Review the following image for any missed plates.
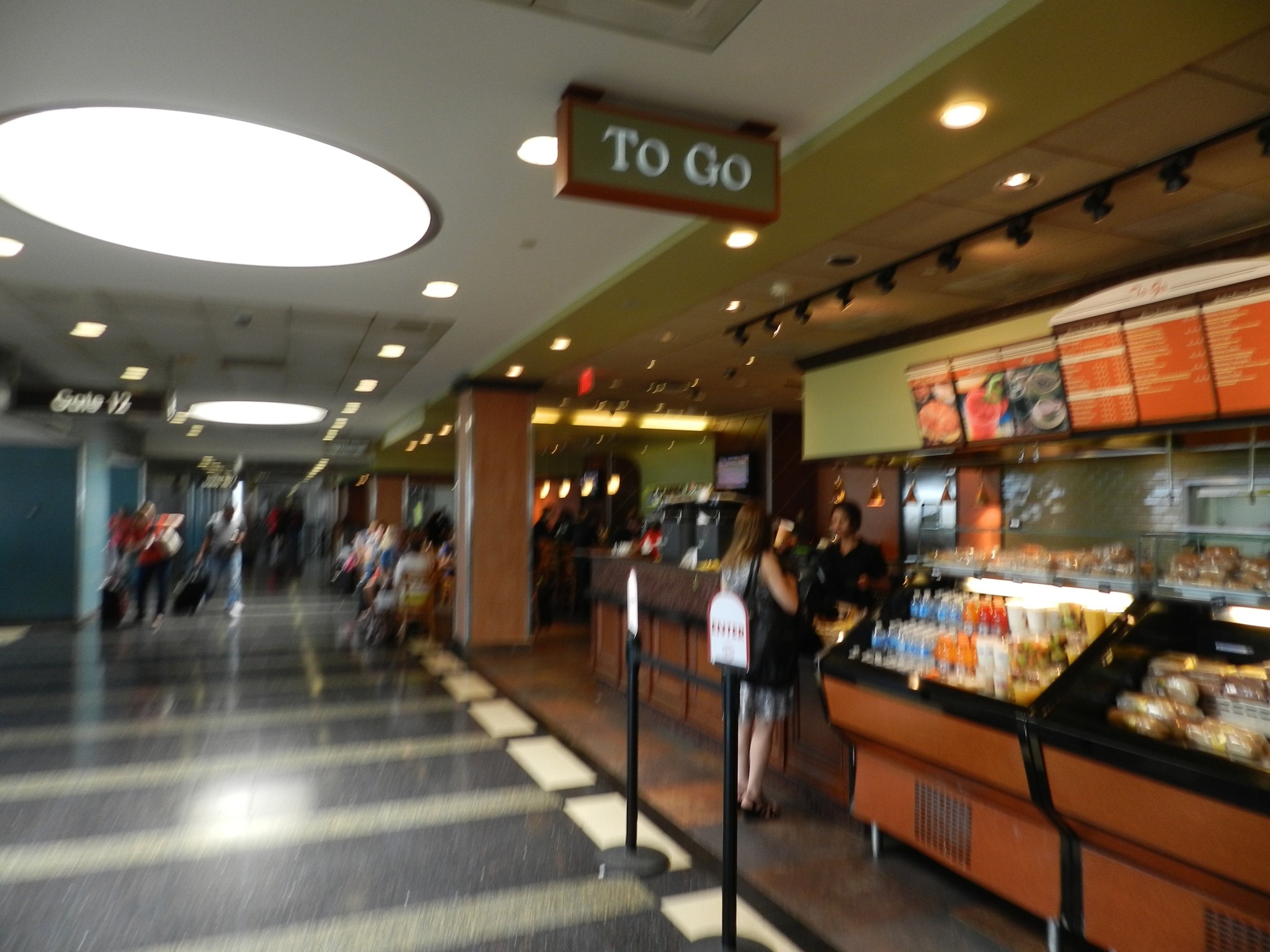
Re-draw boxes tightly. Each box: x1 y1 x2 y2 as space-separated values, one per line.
1030 402 1066 431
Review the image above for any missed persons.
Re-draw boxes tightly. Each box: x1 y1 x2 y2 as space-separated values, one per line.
125 502 186 630
107 502 131 574
266 490 670 639
198 502 248 616
815 502 889 592
716 502 800 814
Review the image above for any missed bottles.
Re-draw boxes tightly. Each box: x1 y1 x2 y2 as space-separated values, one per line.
873 587 1004 687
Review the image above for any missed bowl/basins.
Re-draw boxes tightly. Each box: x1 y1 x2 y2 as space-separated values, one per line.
1026 373 1060 402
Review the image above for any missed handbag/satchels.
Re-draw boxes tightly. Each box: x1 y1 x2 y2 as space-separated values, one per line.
741 549 800 689
159 531 182 555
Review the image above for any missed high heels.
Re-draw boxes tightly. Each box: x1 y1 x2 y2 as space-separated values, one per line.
737 797 780 823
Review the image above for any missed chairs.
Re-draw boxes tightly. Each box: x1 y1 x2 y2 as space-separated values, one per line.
394 570 437 647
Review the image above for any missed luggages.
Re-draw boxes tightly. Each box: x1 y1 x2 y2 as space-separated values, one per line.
102 575 129 630
172 564 209 618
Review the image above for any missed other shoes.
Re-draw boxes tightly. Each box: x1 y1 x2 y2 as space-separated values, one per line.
147 616 164 631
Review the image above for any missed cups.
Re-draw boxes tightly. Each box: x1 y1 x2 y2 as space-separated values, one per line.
974 591 1121 706
965 389 1000 441
1036 400 1063 422
